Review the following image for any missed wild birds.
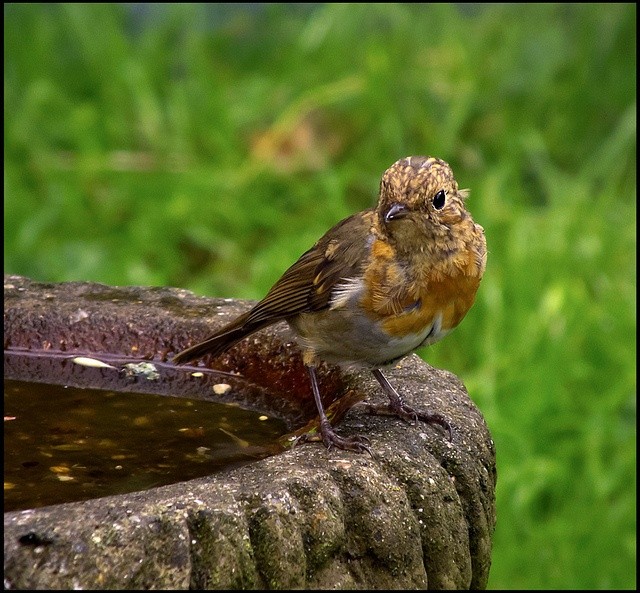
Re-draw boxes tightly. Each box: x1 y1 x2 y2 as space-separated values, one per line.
170 153 487 452
